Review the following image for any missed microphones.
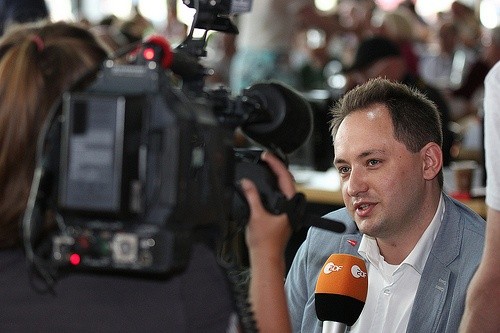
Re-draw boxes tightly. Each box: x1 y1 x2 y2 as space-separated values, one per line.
314 254 368 333
239 82 314 154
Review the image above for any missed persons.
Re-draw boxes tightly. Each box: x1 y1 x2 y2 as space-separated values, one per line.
1 1 500 170
459 60 500 332
283 76 488 333
0 23 298 333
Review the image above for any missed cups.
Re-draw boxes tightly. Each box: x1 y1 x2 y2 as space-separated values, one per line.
455 168 475 192
